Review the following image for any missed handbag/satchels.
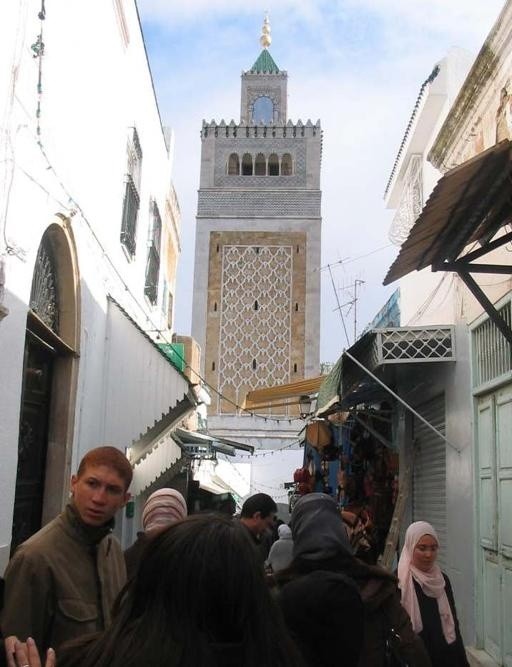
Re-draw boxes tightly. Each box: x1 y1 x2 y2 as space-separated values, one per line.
342 437 390 503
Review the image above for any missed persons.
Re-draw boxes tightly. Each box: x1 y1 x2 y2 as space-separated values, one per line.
0 484 473 667
2 444 136 665
